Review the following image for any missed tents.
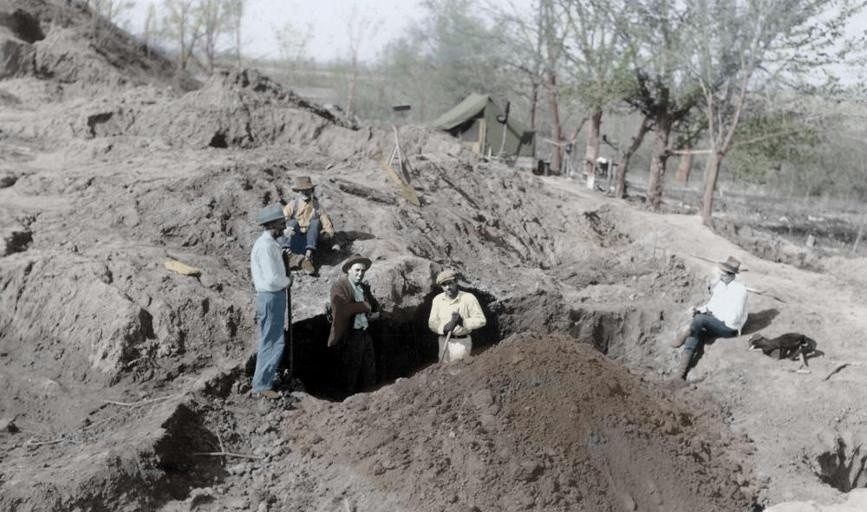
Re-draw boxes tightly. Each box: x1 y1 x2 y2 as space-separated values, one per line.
436 90 537 163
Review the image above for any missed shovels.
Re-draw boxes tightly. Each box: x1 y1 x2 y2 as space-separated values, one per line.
281 251 294 390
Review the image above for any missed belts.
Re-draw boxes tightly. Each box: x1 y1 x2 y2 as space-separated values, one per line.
443 333 468 338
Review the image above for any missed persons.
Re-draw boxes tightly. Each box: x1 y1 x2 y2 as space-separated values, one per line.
428 270 487 364
328 252 381 392
249 203 294 402
672 254 748 380
278 174 341 274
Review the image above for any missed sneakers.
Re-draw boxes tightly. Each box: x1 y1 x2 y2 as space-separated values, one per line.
286 251 318 275
262 390 279 399
671 330 692 348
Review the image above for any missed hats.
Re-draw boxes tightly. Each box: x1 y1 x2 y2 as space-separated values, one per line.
720 255 741 274
342 253 372 274
434 270 460 288
292 176 315 191
254 205 286 226
